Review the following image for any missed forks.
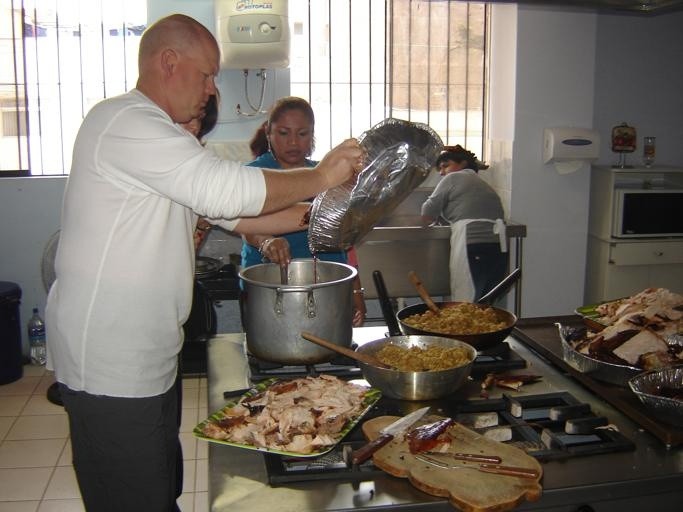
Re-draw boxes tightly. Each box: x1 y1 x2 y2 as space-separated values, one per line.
410 452 541 481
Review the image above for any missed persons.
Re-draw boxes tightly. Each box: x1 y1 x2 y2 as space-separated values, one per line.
421 147 508 304
249 119 367 326
44 14 365 511
240 97 349 312
177 87 220 257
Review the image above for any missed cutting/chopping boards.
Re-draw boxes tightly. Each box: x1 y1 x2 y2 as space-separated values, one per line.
362 413 547 512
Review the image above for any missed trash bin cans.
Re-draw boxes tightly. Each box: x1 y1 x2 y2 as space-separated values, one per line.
0 281 22 386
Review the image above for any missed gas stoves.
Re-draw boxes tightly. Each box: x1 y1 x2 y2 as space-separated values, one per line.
242 334 640 487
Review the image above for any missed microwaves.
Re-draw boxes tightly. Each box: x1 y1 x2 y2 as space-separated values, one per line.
610 186 683 238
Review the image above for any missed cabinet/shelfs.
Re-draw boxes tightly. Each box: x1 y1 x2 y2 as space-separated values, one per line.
586 164 682 304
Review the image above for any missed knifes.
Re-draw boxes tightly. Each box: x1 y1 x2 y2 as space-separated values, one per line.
398 448 505 467
350 404 430 463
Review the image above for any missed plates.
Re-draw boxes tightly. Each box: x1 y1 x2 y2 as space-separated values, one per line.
194 255 225 280
556 296 683 430
191 376 384 461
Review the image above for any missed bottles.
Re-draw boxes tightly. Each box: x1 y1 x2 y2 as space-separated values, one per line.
644 136 657 168
27 306 48 366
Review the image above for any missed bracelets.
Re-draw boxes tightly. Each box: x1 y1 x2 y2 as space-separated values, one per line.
258 238 273 254
353 288 365 296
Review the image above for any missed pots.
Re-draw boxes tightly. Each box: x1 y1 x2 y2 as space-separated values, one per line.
392 267 527 346
354 269 479 403
233 258 358 368
